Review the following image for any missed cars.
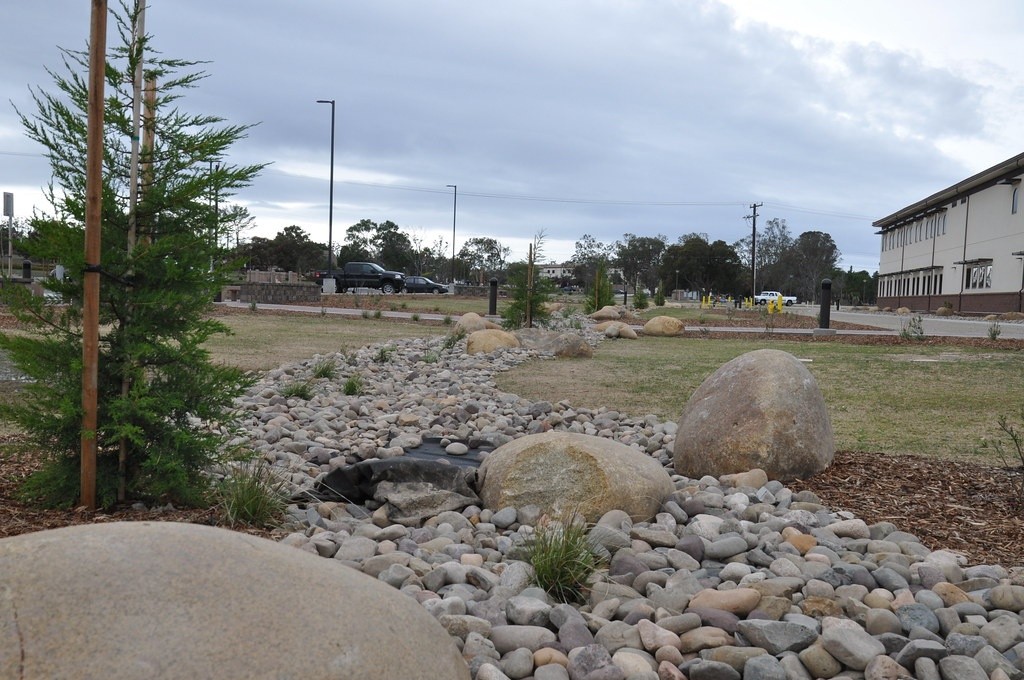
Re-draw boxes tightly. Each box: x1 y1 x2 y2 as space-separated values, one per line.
399 276 449 295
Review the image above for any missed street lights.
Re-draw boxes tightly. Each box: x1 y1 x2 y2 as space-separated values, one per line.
446 185 457 293
315 98 336 295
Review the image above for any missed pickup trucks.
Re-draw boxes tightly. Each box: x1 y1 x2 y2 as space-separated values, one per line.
315 261 406 295
754 291 797 306
